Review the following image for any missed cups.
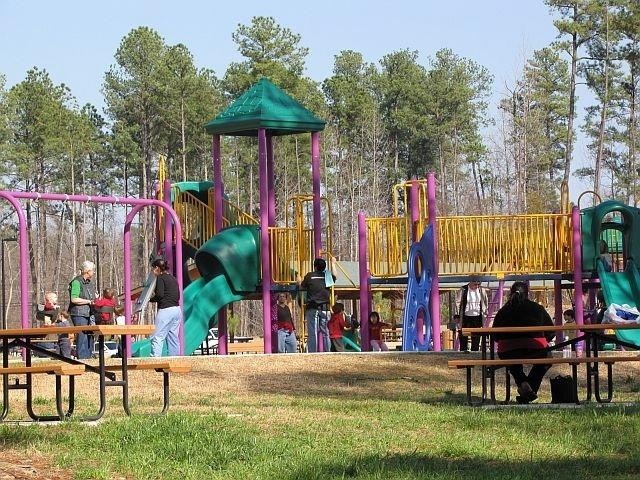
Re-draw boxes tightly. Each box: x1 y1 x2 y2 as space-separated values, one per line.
575 344 582 358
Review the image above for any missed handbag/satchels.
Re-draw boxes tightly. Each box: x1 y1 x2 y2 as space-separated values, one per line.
549 375 579 403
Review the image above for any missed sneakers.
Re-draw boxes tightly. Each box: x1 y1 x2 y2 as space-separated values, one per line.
111 352 123 358
516 382 538 404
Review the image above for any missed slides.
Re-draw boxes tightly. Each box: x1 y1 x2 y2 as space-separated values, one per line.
131 224 261 357
580 201 640 347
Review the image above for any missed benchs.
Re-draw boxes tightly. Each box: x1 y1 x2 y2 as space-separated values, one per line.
0 365 87 421
448 355 640 406
26 363 194 416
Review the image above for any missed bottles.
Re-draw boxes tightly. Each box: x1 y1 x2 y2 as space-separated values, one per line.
561 335 571 359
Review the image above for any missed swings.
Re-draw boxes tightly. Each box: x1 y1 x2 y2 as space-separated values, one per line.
82 204 116 325
35 200 66 325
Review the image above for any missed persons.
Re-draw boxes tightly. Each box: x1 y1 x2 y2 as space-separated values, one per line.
600 239 613 272
454 314 461 330
32 259 139 360
302 258 336 353
148 257 181 356
369 311 392 351
277 292 299 353
327 303 353 352
490 281 556 405
453 275 489 351
563 309 577 351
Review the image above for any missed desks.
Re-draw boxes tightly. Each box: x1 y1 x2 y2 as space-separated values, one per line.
462 323 640 405
0 324 170 421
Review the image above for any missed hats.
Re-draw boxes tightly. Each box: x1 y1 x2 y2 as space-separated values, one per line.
81 260 96 275
